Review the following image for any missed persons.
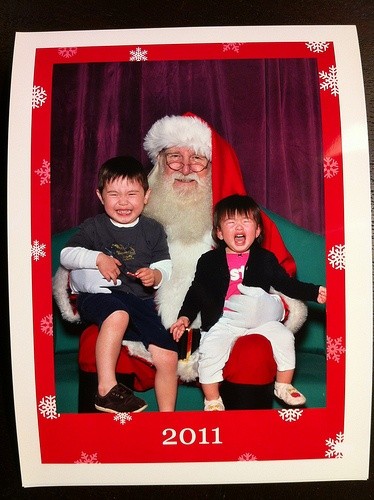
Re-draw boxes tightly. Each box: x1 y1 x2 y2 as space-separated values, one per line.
172 192 327 412
51 108 308 413
59 156 183 416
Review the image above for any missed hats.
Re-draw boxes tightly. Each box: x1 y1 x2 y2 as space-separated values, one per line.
143 113 246 204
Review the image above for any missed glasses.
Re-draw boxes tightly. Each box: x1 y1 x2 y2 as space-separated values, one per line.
164 150 209 173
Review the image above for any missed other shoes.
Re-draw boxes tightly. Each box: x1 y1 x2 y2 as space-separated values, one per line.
204 400 224 411
272 383 307 405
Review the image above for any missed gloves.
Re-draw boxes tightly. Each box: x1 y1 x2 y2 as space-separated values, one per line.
219 282 285 328
67 266 122 294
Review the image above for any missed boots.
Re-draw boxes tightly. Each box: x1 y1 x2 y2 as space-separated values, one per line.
78 369 134 413
221 379 274 410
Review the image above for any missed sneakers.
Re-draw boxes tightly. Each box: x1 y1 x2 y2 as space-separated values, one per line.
95 382 148 413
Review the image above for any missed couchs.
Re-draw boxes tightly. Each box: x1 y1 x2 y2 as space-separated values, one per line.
49 207 327 414
